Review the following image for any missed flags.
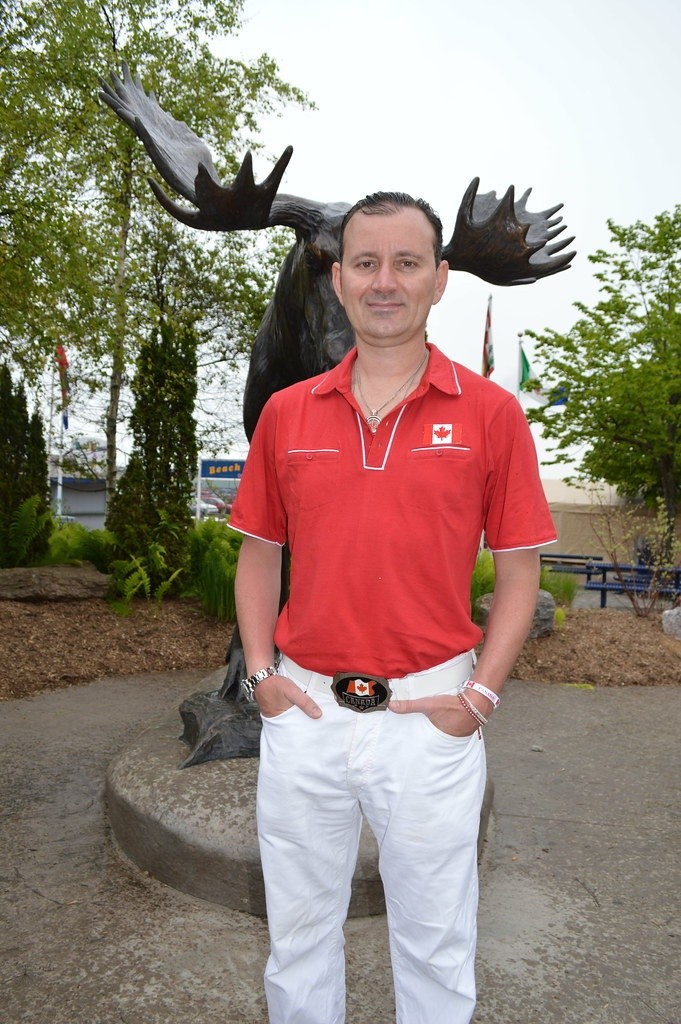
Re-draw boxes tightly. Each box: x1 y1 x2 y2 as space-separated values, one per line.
55 344 68 428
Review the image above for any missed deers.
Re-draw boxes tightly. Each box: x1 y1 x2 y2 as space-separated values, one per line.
88 56 578 770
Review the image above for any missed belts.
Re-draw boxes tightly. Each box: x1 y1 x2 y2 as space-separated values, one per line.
280 653 474 713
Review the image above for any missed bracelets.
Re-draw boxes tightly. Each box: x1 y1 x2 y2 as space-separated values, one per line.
242 666 276 703
464 681 500 708
457 693 487 727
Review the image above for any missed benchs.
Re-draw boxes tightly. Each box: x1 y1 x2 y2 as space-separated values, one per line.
539 554 681 608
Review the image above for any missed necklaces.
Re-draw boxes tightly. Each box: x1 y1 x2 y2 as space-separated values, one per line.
351 354 427 432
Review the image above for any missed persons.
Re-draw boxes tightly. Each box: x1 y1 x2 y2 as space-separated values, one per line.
224 194 558 1024
